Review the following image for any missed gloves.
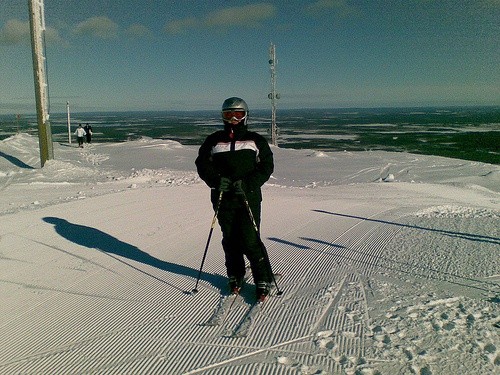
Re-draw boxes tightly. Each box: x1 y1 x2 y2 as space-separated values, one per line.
219 177 230 192
233 180 245 194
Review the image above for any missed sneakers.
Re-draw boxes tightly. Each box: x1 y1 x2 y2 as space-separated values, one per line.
229 275 245 294
256 281 271 298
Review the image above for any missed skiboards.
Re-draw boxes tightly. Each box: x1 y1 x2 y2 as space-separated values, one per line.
229 273 282 338
202 266 253 327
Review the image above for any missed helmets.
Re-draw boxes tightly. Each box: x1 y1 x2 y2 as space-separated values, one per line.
221 97 249 126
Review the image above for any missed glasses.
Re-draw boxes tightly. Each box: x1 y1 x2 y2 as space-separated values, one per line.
221 109 247 121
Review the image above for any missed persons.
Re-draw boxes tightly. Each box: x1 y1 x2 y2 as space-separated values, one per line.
195 97 276 301
73 124 86 146
84 123 92 143
